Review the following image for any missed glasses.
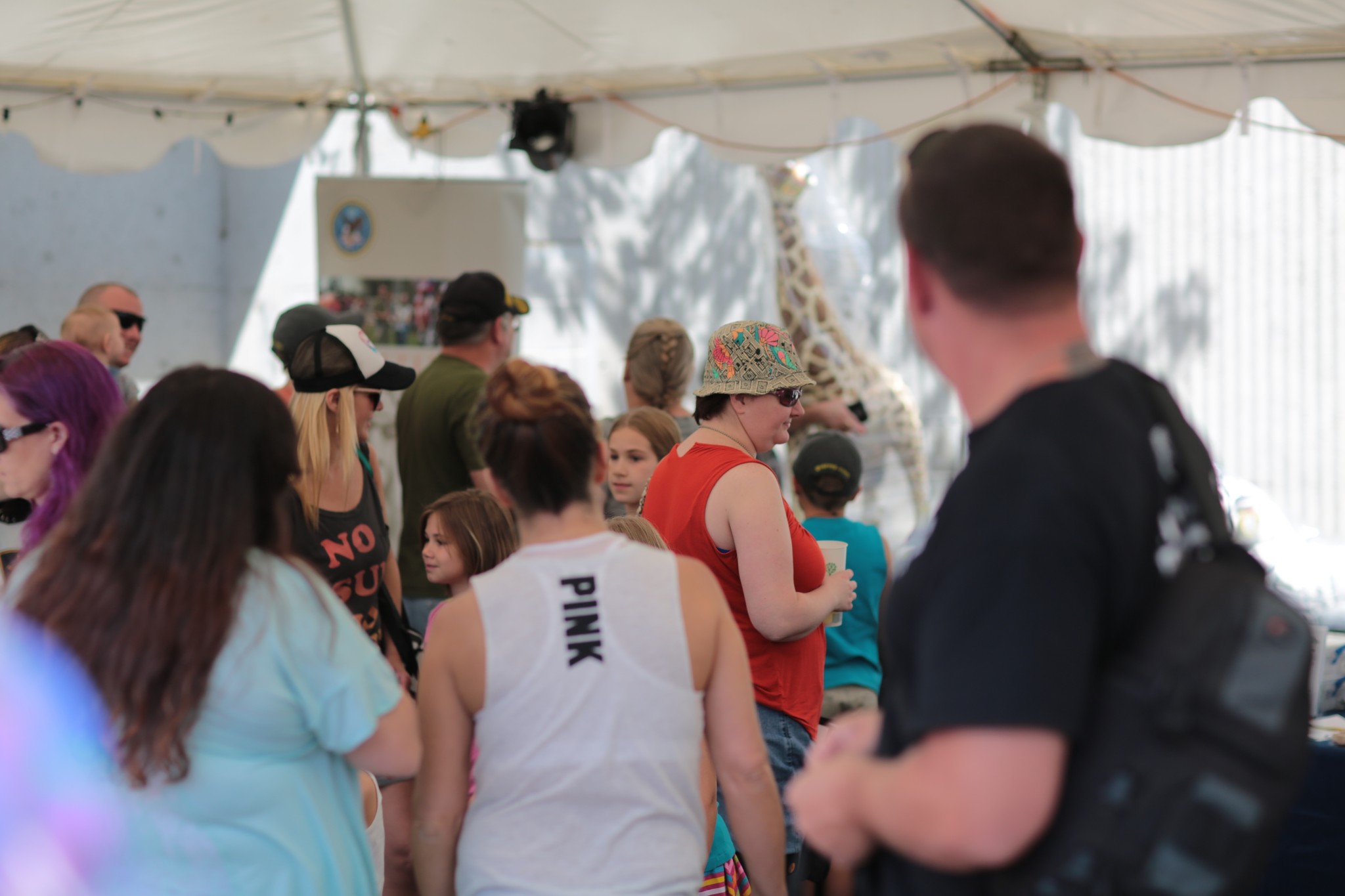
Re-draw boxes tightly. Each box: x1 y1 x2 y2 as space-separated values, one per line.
1 418 56 450
112 309 144 331
771 387 804 407
352 389 380 410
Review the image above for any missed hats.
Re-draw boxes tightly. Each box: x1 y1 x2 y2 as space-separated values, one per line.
271 303 335 368
287 322 416 393
693 321 817 397
438 272 530 319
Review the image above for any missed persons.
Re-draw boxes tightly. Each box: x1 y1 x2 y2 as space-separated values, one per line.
781 123 1245 896
0 272 899 896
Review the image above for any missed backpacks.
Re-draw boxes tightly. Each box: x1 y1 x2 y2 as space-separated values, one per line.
1011 374 1315 896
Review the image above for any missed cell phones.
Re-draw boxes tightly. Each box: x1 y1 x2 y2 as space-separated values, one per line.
849 401 867 424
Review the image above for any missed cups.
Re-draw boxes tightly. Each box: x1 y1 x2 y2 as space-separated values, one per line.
815 540 848 628
